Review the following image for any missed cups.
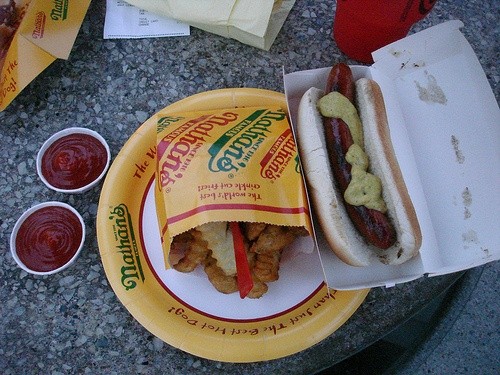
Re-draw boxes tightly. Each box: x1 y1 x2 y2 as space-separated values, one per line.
329 0 433 62
34 127 112 193
8 202 87 275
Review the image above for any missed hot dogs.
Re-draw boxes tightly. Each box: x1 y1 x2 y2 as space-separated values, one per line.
297 62 423 268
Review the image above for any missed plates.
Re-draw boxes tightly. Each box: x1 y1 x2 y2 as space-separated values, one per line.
97 88 369 365
